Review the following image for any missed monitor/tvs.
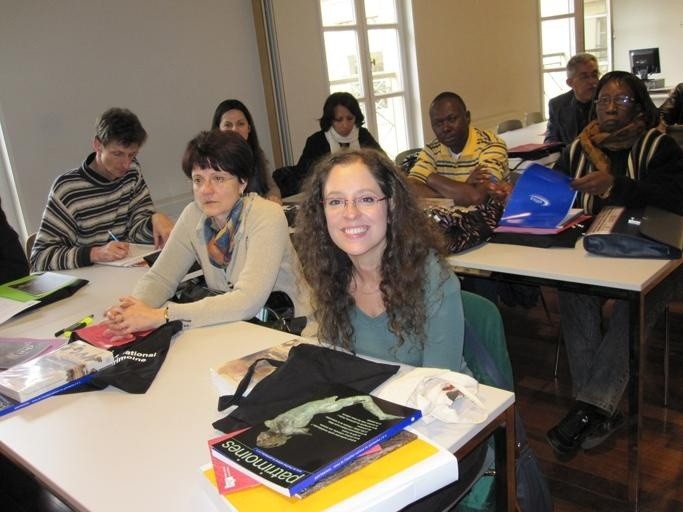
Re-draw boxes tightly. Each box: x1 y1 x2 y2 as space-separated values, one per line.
628 48 660 80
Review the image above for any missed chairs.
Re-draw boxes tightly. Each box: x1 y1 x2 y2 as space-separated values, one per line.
391 146 424 168
24 232 37 262
456 288 552 511
524 112 544 126
495 116 523 135
268 164 307 199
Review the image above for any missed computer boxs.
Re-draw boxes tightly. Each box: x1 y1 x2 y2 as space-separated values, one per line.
641 79 665 89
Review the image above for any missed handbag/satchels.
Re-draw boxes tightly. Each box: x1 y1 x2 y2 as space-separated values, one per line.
175 279 296 335
50 319 183 399
505 433 555 512
584 198 682 258
217 344 399 440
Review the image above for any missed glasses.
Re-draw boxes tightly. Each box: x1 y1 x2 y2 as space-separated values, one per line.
185 175 238 185
318 196 389 208
592 95 637 106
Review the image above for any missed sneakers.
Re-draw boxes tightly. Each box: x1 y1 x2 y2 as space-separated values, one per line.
545 405 592 451
580 410 626 449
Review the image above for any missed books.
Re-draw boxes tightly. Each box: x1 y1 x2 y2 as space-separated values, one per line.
406 163 593 236
1 271 90 323
1 320 151 417
203 345 458 512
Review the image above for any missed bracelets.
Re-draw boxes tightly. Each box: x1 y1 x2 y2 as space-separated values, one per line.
164 307 169 324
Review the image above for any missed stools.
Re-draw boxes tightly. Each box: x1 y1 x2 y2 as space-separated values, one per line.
552 289 671 412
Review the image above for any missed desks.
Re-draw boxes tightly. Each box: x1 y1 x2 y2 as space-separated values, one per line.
0 194 303 334
444 237 682 510
0 299 515 510
498 121 562 174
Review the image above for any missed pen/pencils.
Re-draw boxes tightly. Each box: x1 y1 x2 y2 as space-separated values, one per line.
54 313 94 338
107 230 119 241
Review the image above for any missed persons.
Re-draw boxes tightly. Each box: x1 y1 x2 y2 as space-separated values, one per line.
658 82 683 134
292 147 496 511
29 106 175 271
213 100 283 207
544 53 601 171
406 91 518 363
545 71 683 461
103 128 319 343
295 92 391 176
